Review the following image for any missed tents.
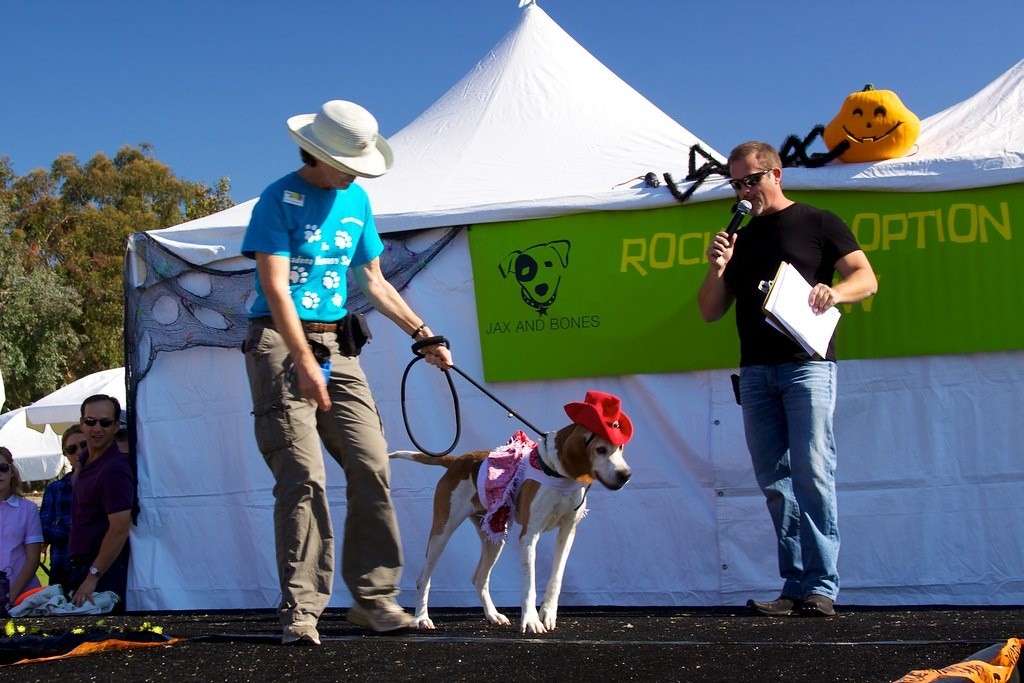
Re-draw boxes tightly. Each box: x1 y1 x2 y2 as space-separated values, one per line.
126 1 1024 612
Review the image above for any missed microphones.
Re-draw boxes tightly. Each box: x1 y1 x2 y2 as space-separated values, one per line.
714 199 752 258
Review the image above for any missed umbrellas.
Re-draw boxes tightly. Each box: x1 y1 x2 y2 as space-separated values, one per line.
26 368 127 435
0 404 72 482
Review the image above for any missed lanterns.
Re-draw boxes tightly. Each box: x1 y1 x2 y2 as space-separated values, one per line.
823 84 920 162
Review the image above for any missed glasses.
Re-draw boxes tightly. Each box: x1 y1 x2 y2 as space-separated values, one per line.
0 463 13 473
64 440 87 454
83 417 116 427
728 168 775 190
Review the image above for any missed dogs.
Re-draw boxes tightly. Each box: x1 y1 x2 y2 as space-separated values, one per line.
388 390 631 634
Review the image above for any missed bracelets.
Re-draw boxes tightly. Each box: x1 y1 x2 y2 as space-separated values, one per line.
411 324 427 339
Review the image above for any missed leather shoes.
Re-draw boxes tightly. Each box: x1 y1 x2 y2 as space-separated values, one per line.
800 594 837 615
751 596 805 614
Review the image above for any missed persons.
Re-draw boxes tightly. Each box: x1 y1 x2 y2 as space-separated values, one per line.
0 446 44 607
698 141 878 615
241 100 453 645
40 393 130 611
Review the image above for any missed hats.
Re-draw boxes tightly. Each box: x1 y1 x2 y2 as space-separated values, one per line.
564 391 633 445
287 99 393 177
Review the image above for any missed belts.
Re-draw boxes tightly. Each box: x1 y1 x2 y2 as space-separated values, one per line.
249 313 339 334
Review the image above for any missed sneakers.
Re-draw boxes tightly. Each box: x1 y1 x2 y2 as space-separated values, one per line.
345 601 417 633
281 613 322 646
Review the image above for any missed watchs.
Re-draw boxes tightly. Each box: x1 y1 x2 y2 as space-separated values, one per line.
90 567 103 577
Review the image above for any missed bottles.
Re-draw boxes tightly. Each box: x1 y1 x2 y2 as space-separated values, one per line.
0 565 13 618
